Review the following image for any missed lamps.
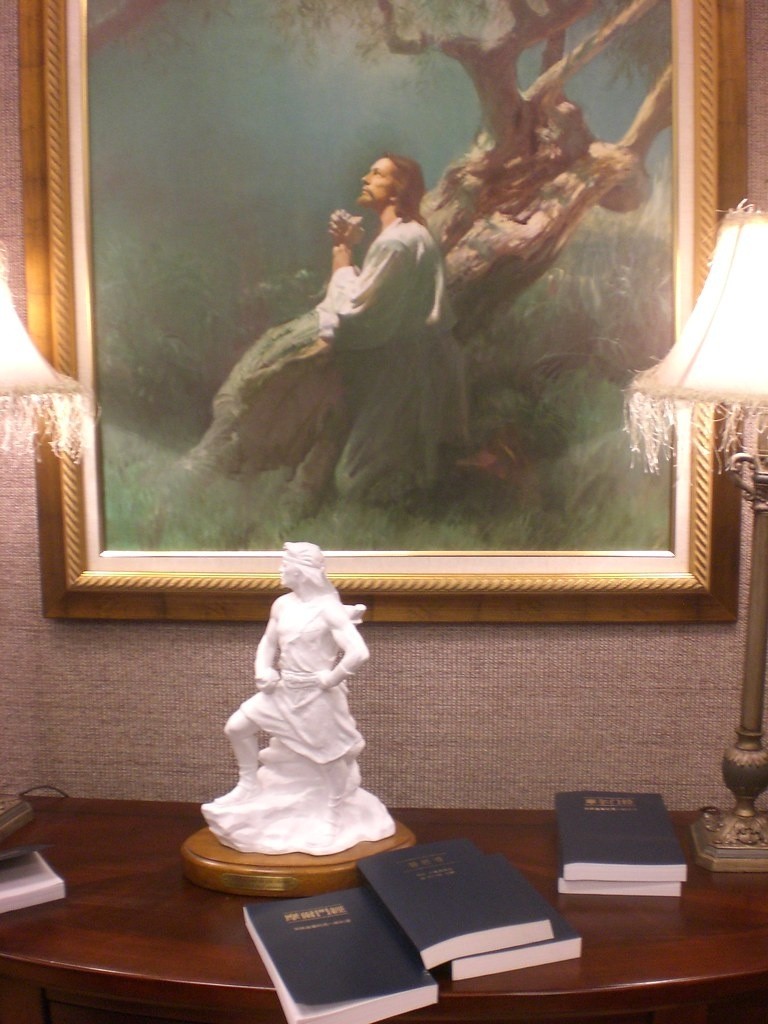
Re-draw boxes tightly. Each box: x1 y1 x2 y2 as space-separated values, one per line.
622 195 768 869
0 260 103 843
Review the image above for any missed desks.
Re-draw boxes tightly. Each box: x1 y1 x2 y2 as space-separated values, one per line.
0 798 768 1021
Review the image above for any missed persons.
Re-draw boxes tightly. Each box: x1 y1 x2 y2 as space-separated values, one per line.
215 541 372 846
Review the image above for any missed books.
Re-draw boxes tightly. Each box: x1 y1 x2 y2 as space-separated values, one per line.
241 888 439 1024
553 791 688 897
0 843 67 914
357 838 583 981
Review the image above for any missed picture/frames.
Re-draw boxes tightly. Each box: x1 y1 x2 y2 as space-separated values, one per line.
18 1 754 627
